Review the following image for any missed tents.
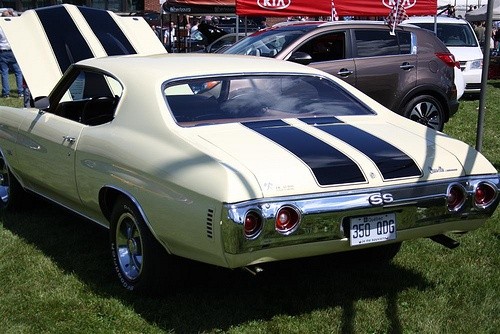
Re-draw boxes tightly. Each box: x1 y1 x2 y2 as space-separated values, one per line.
161 0 247 53
235 0 437 44
465 0 500 23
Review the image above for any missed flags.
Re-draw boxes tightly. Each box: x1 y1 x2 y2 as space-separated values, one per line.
386 3 409 28
332 0 339 21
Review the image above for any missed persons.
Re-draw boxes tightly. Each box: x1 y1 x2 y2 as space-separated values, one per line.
151 19 203 43
0 8 31 108
258 18 270 29
473 21 500 56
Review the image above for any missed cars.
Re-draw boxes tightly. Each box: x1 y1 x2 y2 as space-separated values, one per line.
0 4 500 296
217 19 460 132
399 16 483 94
191 32 254 53
